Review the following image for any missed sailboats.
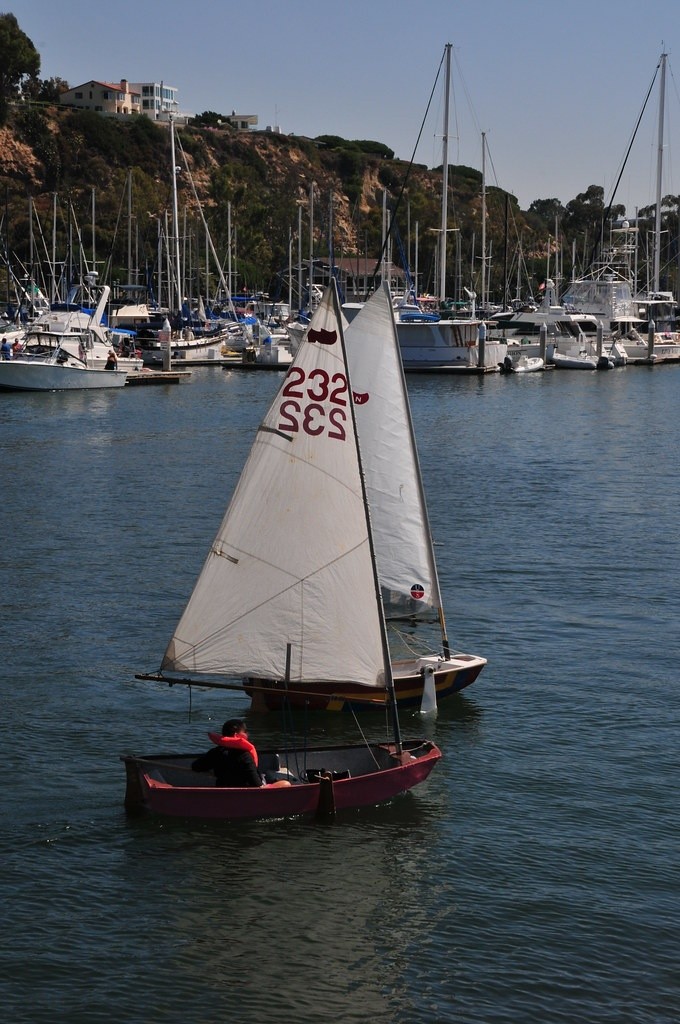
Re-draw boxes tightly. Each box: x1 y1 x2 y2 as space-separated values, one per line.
240 265 490 712
120 276 445 823
1 41 680 393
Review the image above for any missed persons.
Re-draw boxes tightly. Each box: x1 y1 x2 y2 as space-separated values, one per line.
79 343 87 362
1 338 10 360
118 342 123 356
13 338 23 356
192 719 263 787
29 305 33 317
104 350 116 370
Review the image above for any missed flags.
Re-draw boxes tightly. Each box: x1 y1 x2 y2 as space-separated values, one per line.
539 282 545 290
